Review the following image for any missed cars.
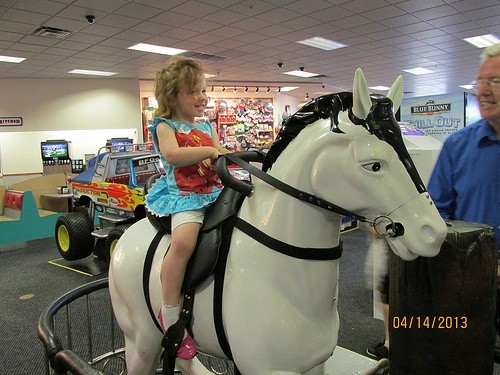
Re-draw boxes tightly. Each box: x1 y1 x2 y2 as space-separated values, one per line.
54 142 166 269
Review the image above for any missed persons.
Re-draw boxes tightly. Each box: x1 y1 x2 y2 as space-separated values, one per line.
427 43 500 240
366 272 389 359
144 56 231 360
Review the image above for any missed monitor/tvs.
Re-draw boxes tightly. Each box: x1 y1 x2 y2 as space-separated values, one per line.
110 138 133 152
41 140 68 161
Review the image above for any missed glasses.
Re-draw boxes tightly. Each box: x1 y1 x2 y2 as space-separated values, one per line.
472 78 500 88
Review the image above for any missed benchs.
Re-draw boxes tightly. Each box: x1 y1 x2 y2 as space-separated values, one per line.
0 190 74 221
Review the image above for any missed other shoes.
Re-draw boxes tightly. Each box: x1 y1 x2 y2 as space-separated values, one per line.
366 340 389 358
157 307 198 361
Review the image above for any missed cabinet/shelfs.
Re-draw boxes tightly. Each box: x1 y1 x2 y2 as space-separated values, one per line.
143 97 275 150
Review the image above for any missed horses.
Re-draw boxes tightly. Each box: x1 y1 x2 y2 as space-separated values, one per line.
108 68 447 375
